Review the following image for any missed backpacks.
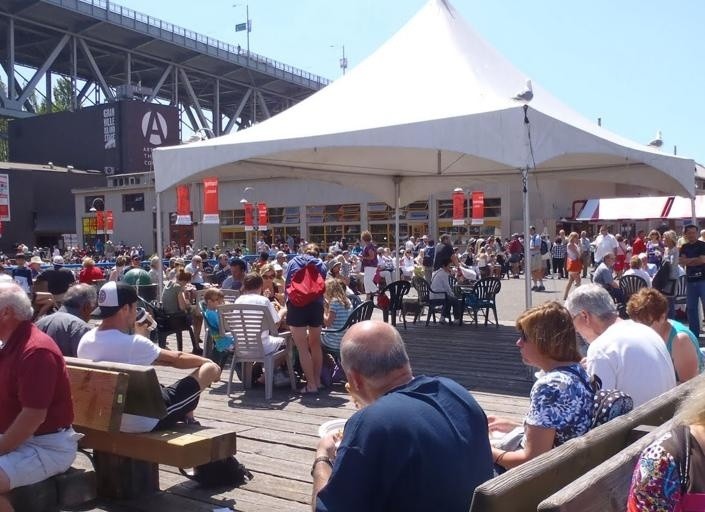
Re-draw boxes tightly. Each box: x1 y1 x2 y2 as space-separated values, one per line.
178 419 254 491
550 366 635 429
533 235 549 255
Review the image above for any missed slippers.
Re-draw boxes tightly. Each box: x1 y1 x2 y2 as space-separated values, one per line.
296 386 317 395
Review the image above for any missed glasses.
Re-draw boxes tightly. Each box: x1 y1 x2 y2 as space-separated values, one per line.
262 274 275 280
407 252 411 253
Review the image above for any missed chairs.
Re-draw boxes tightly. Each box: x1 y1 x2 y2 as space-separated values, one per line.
657 275 688 320
589 269 595 283
132 282 193 352
376 279 411 330
618 275 648 308
411 273 501 329
195 274 376 401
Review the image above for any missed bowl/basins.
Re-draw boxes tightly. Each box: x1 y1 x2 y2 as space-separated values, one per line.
318 418 348 466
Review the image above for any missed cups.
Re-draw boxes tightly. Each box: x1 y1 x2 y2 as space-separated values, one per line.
136 307 158 333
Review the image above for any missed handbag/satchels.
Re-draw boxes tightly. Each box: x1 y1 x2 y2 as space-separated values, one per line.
285 262 326 309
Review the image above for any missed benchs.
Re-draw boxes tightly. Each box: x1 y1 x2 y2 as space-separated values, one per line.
0 355 236 512
468 370 705 512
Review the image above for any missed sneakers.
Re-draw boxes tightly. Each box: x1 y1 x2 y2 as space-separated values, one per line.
535 285 545 292
531 286 538 290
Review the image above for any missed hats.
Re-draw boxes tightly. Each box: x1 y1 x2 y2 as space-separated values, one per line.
88 280 139 318
52 255 65 267
274 264 283 271
29 256 45 265
328 257 342 272
275 250 287 258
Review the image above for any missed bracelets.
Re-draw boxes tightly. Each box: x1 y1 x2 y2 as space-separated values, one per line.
310 456 334 475
495 450 510 466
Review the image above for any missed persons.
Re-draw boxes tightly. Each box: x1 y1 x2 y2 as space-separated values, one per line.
1 235 362 394
0 281 84 512
531 222 704 336
563 282 677 405
76 281 221 434
482 300 593 479
625 370 705 511
310 319 492 512
354 230 523 325
626 288 705 384
